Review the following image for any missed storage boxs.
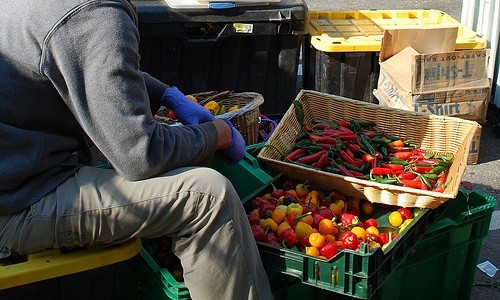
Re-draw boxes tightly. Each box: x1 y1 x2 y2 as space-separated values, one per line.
0 238 142 300
139 152 273 300
257 89 478 210
256 186 497 300
131 0 309 115
372 26 491 126
256 200 457 300
301 9 487 105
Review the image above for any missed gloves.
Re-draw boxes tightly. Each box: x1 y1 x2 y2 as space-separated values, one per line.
161 85 216 126
214 118 246 165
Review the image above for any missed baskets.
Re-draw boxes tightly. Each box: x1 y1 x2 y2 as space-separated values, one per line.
152 91 264 146
257 89 478 210
240 172 449 299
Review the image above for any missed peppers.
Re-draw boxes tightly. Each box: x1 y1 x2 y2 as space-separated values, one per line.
282 118 453 193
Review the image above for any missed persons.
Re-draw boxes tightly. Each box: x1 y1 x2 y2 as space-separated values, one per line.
0 0 274 300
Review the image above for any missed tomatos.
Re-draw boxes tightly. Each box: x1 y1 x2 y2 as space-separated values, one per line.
246 181 425 259
